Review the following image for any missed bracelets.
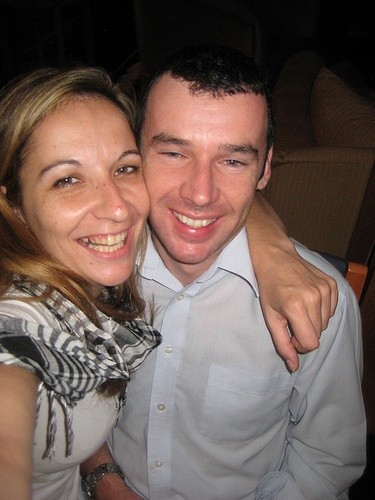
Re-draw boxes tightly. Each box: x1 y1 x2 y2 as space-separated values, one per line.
78 462 126 500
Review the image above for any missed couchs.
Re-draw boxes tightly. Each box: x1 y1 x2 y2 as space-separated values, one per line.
257 47 375 268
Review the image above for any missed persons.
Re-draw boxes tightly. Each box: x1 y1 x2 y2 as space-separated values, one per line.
75 57 370 500
0 66 340 500
